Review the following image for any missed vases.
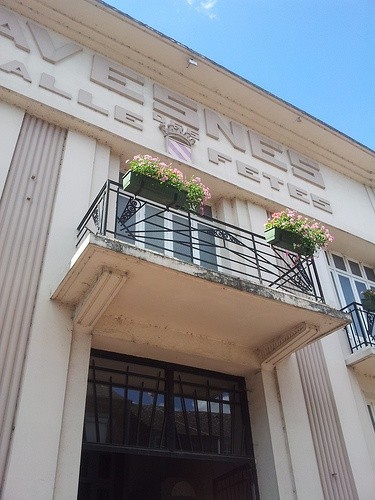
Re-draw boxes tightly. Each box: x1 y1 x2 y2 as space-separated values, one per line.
263 227 314 256
121 169 188 209
361 299 375 312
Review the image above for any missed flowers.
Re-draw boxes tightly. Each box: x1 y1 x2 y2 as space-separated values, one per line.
125 153 212 217
360 289 375 300
264 207 334 261
